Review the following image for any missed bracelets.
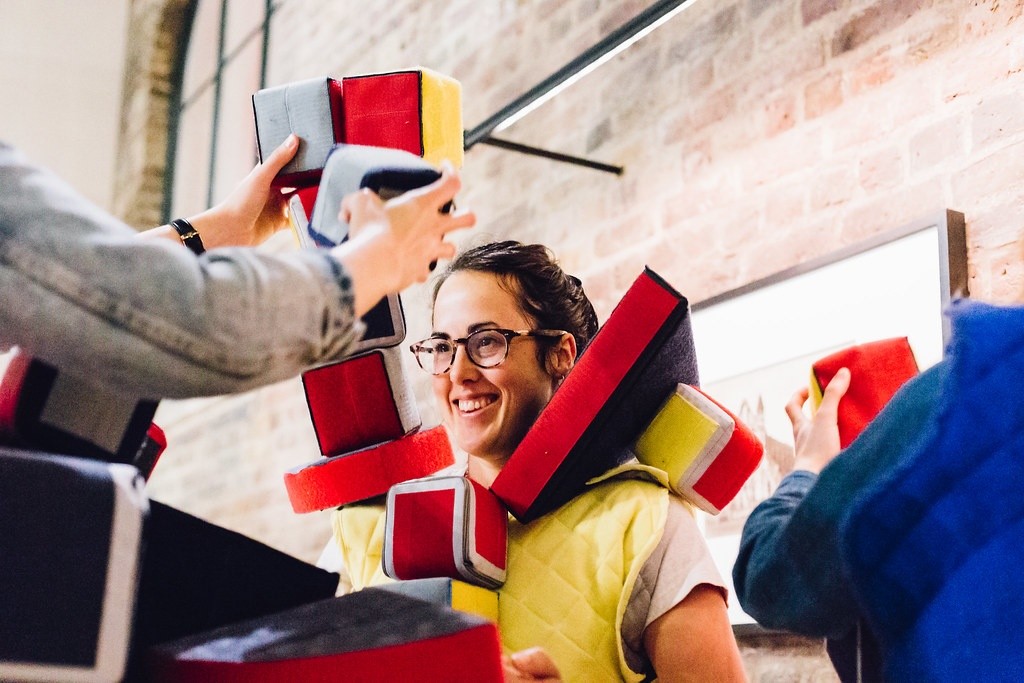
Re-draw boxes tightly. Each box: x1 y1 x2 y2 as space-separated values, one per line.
170 219 207 254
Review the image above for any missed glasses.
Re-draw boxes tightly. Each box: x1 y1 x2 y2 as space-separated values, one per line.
409 329 568 377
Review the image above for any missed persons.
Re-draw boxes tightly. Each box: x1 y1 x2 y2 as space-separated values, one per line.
309 240 751 682
0 123 478 683
732 282 1024 683
